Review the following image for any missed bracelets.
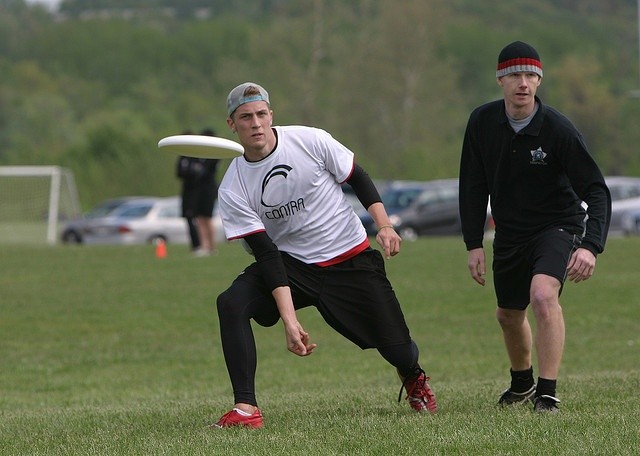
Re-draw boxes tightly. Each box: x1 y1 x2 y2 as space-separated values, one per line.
377 224 393 231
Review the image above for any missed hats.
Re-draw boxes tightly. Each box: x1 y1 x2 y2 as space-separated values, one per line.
226 81 272 118
495 40 546 78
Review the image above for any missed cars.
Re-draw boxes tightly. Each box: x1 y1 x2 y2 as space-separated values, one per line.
356 187 427 233
82 196 223 246
60 194 135 239
371 188 485 240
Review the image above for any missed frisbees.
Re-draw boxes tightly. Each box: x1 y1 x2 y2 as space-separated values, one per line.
158 135 244 159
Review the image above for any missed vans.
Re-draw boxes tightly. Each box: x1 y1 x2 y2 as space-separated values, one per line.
585 175 640 238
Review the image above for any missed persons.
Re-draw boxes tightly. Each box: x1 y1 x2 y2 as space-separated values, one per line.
211 80 438 428
186 128 218 257
459 40 612 415
175 127 200 251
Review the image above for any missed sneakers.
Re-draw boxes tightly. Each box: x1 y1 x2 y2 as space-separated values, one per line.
398 369 440 416
534 394 562 413
209 406 266 429
494 383 538 407
